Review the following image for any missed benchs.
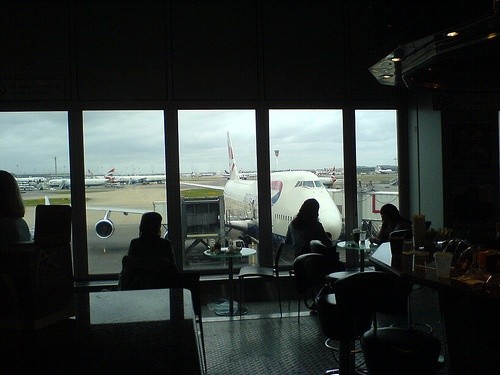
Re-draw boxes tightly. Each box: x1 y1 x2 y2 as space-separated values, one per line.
199 270 295 299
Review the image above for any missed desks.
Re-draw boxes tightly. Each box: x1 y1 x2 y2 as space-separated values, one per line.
368 242 500 375
336 240 381 271
204 247 257 316
66 287 206 375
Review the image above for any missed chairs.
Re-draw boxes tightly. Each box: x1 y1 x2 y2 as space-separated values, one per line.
293 253 442 375
234 243 284 316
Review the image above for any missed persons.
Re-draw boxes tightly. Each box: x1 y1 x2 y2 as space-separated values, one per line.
369 203 400 243
128 212 178 274
285 199 333 254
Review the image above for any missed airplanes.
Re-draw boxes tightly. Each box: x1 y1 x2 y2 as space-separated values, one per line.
45 130 398 244
14 168 166 188
375 165 394 174
221 166 338 187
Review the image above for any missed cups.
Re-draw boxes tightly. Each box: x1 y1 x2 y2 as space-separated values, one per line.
435 253 452 278
390 230 404 254
353 229 360 242
413 215 425 250
360 231 366 242
